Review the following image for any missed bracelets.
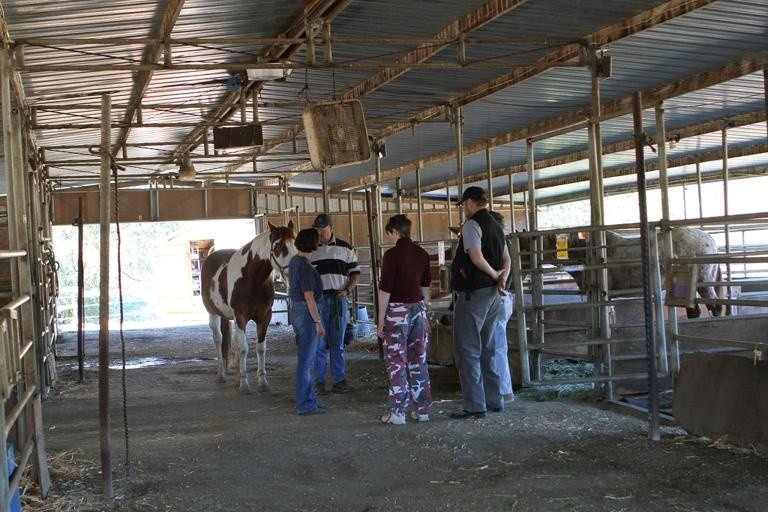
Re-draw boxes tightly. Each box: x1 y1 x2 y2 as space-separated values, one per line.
313 319 322 324
345 287 350 295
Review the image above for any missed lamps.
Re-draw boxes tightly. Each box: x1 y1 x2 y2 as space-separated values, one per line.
178 151 197 182
370 135 389 159
247 58 283 81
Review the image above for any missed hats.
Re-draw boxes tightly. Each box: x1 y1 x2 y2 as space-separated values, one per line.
311 213 331 228
456 187 486 206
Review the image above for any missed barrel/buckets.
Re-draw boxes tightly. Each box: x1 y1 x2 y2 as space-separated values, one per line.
6 444 22 511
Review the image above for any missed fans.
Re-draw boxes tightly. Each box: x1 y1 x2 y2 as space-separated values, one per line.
303 64 371 171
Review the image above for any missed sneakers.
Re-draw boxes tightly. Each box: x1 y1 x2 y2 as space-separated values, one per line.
299 380 354 416
380 392 514 425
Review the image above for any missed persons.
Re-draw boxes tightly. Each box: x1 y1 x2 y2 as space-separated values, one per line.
304 213 364 394
288 228 334 414
375 214 431 424
485 210 516 400
446 186 513 419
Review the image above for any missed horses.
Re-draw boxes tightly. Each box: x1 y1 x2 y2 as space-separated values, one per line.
202 220 300 394
514 224 724 319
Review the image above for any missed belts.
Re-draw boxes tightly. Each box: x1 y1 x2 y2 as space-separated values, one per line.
458 283 498 301
